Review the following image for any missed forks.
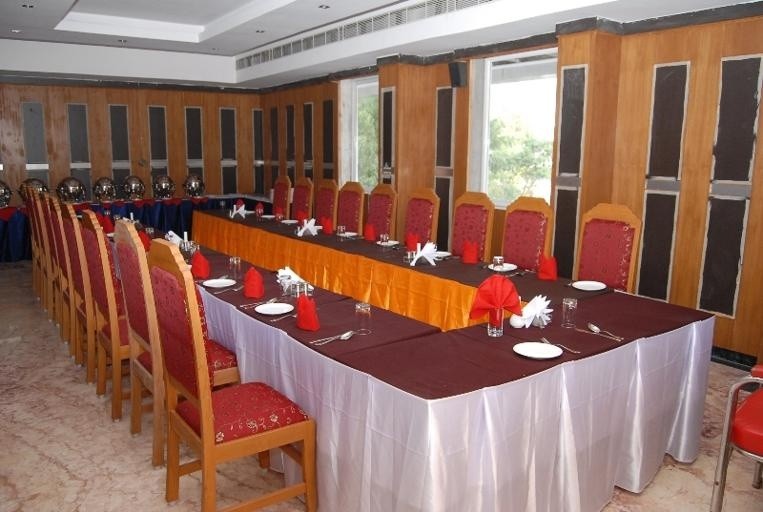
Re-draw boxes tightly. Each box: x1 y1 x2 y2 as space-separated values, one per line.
540 337 581 354
505 270 528 278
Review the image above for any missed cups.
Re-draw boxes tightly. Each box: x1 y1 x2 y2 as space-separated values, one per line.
337 226 345 242
144 227 371 337
403 250 416 263
488 309 503 338
287 281 309 297
380 234 389 251
255 208 262 222
561 298 577 328
276 215 283 223
493 255 505 278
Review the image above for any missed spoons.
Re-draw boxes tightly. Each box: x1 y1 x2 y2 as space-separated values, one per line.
315 330 355 346
587 323 624 340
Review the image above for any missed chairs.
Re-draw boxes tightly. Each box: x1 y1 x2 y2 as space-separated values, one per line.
0 197 209 262
709 364 762 512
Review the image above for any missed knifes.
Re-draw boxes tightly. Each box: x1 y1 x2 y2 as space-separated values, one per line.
575 328 622 342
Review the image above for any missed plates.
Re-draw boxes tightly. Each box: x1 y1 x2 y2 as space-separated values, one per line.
261 214 277 222
513 341 564 360
338 230 358 237
280 219 298 225
488 263 518 273
570 280 606 291
378 239 400 248
435 251 451 260
